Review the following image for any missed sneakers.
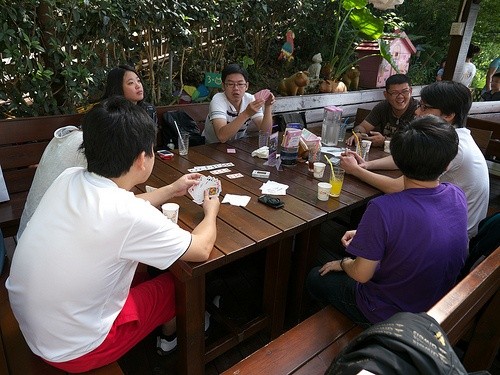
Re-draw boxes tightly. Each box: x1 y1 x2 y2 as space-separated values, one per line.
157 310 210 356
205 294 227 315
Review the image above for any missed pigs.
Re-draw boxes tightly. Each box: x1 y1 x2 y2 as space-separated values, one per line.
277 66 360 97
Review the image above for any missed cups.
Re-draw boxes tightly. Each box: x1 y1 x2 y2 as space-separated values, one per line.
308 147 320 172
321 106 343 146
162 203 180 224
281 144 299 167
384 140 391 153
357 140 372 161
259 131 269 148
318 182 332 201
338 124 346 141
178 134 189 155
329 167 346 197
313 162 326 179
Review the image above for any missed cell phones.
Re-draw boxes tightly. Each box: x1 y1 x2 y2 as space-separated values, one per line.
157 150 174 157
258 194 284 208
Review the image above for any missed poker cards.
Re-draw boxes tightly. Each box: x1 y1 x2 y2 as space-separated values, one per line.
210 168 231 175
226 173 244 180
227 149 235 153
187 162 235 173
254 89 271 101
187 175 221 205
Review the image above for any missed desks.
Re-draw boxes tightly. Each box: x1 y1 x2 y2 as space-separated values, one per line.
466 101 500 160
129 120 404 375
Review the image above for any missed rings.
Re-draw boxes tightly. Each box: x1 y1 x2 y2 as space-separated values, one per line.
349 137 351 140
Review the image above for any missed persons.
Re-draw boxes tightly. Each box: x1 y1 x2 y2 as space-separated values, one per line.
305 115 469 327
0 96 220 375
103 66 153 119
345 74 421 146
436 44 500 102
340 80 489 240
201 64 275 143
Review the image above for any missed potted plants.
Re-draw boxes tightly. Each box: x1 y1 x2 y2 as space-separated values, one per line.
319 0 400 93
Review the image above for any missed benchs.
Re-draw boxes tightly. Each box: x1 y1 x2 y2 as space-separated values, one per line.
0 232 126 375
219 241 500 375
0 85 426 279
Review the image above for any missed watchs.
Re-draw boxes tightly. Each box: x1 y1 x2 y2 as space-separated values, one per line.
354 130 362 133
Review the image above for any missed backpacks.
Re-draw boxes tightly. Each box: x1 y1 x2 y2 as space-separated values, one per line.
163 110 205 149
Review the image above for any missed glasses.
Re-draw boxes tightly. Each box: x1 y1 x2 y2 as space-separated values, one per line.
420 100 440 111
224 82 248 88
386 88 410 97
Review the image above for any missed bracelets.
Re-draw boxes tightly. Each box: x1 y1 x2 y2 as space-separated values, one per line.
366 162 368 169
385 135 388 141
341 257 351 271
